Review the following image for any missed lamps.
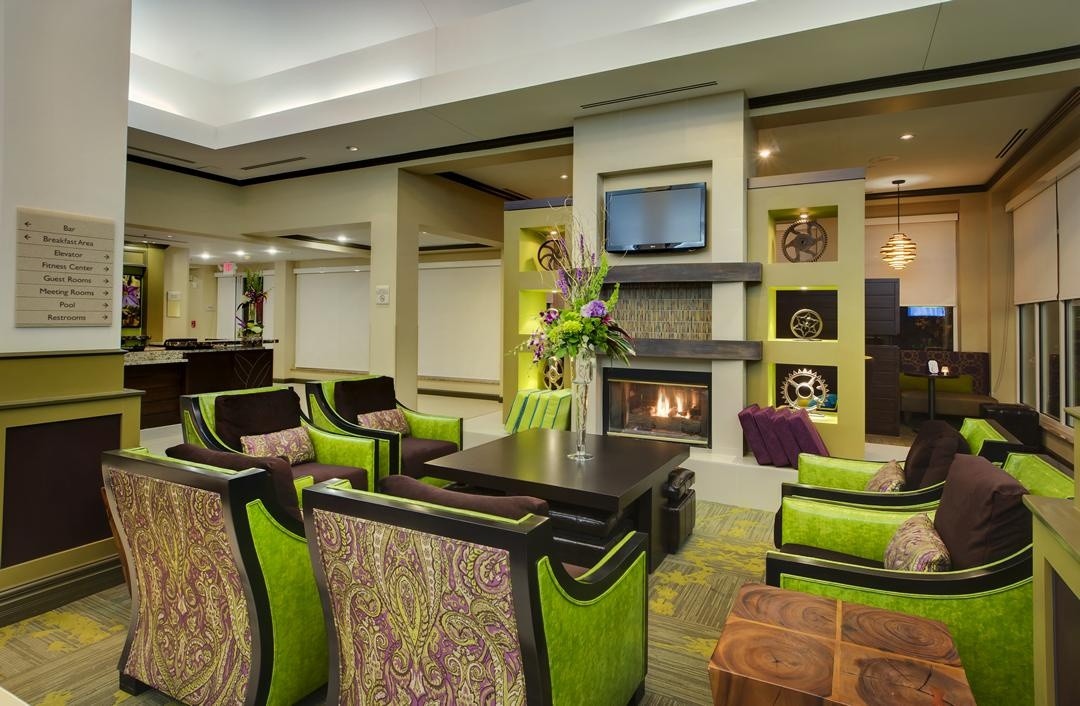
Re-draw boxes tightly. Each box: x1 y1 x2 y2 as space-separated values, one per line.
879 180 917 270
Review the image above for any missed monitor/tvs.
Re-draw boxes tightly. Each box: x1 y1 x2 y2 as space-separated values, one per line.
604 181 706 252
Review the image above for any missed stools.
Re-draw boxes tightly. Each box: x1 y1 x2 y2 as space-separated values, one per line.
979 403 1039 453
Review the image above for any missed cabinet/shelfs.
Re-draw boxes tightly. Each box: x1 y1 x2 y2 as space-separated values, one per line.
503 205 572 423
748 178 866 459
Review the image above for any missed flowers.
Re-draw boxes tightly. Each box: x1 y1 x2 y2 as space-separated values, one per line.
506 226 640 426
236 269 272 337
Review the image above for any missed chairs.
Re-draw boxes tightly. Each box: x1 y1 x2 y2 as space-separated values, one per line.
302 476 649 706
179 385 379 507
766 450 1074 706
775 417 1024 548
99 444 353 706
305 376 463 489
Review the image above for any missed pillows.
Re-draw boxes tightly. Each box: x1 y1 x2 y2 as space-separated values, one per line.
865 458 906 492
884 511 952 573
357 408 409 436
240 427 315 466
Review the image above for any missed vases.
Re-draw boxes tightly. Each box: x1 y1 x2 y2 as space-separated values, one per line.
567 336 595 461
245 334 262 345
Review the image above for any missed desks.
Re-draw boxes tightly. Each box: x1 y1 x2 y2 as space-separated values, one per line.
904 371 960 432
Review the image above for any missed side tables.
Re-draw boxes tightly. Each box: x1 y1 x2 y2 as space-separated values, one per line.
709 580 976 705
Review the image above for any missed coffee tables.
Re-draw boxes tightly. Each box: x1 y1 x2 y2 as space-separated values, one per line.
422 426 696 573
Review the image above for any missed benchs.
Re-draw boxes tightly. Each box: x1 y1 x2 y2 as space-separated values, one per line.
900 350 999 417
